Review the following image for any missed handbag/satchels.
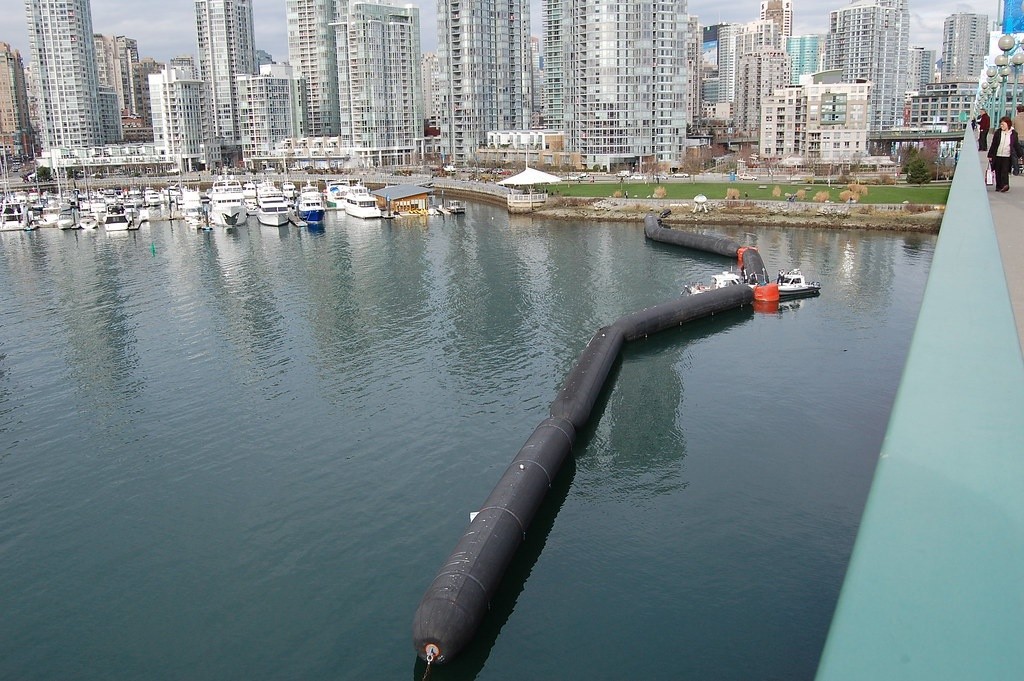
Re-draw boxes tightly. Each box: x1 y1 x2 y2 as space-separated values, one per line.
985 161 994 185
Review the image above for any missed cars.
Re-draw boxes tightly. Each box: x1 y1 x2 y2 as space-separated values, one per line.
442 165 758 184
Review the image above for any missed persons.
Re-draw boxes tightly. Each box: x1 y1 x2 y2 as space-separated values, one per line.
976 108 990 151
1012 104 1024 149
987 117 1023 193
739 265 800 289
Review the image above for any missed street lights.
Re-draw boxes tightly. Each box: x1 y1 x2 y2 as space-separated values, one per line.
973 33 1024 131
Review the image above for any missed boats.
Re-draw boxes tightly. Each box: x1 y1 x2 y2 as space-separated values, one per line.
446 201 465 213
683 266 743 297
0 132 385 233
746 268 822 299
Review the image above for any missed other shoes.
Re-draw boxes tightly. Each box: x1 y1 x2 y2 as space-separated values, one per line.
996 185 1009 192
